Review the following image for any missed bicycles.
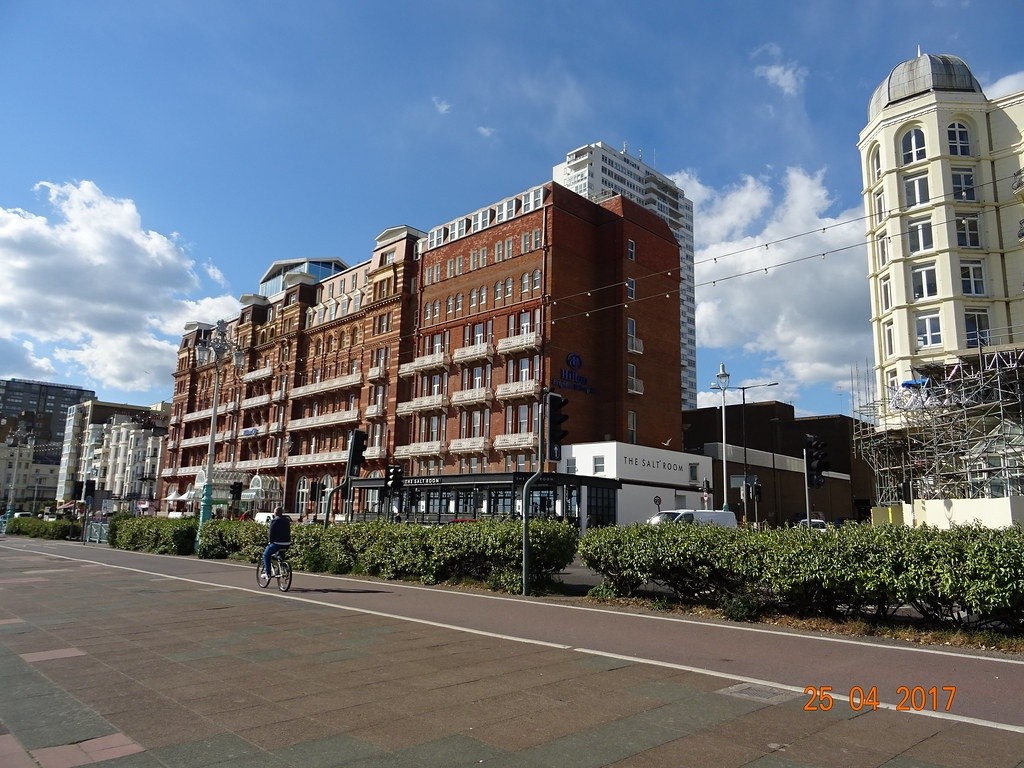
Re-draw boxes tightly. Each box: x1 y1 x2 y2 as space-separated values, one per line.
254 536 293 592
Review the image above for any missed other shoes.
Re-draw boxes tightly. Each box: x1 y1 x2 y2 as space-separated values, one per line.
260 573 272 580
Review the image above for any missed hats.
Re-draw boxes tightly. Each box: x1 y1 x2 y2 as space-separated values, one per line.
274 506 282 513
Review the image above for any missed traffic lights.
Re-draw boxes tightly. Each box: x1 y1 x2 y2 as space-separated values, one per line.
406 487 413 501
412 487 422 508
393 466 403 497
805 438 828 490
747 483 753 500
755 483 761 502
545 391 571 464
229 482 242 500
310 481 326 502
384 464 396 497
347 428 369 478
341 478 351 500
83 479 96 501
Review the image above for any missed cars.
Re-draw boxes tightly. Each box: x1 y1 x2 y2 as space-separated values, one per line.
832 516 850 528
798 519 827 534
0 511 32 533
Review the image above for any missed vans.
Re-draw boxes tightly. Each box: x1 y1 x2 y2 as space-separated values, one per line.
646 509 737 530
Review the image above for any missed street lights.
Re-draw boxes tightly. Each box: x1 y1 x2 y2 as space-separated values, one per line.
192 319 247 555
712 360 732 511
4 427 36 536
709 383 781 524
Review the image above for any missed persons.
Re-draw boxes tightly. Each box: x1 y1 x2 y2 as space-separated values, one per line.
260 505 296 581
